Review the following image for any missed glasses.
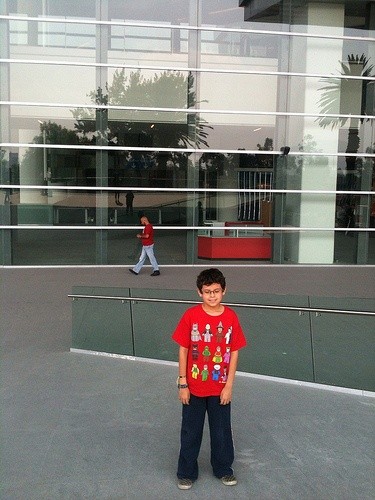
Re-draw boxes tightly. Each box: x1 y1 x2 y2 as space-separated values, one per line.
203 288 224 294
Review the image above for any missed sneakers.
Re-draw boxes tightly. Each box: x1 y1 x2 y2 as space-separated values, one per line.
220 474 237 485
178 478 192 490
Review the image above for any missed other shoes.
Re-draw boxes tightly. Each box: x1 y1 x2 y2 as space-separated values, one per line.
151 270 160 276
129 268 138 275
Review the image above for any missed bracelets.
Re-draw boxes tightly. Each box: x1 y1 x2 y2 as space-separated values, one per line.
178 376 186 379
177 383 188 389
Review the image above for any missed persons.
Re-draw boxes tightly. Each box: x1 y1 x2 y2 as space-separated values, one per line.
115 183 135 215
194 200 205 227
171 268 247 490
41 178 49 196
129 217 160 276
5 181 10 202
